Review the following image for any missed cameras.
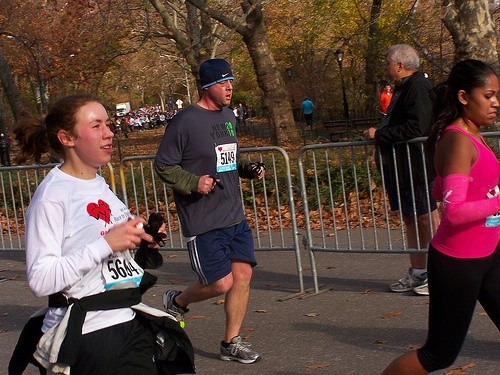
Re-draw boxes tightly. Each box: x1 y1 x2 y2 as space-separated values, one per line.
134 213 163 247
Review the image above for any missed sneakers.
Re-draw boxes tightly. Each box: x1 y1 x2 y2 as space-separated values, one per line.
413 278 429 295
163 290 189 322
390 268 427 292
219 335 261 363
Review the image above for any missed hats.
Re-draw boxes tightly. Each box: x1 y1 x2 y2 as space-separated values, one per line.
199 59 234 89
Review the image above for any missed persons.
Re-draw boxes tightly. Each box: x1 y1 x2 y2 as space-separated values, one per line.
380 60 500 375
23 94 167 375
360 44 440 294
162 59 267 363
379 83 394 118
302 97 315 127
110 104 247 138
